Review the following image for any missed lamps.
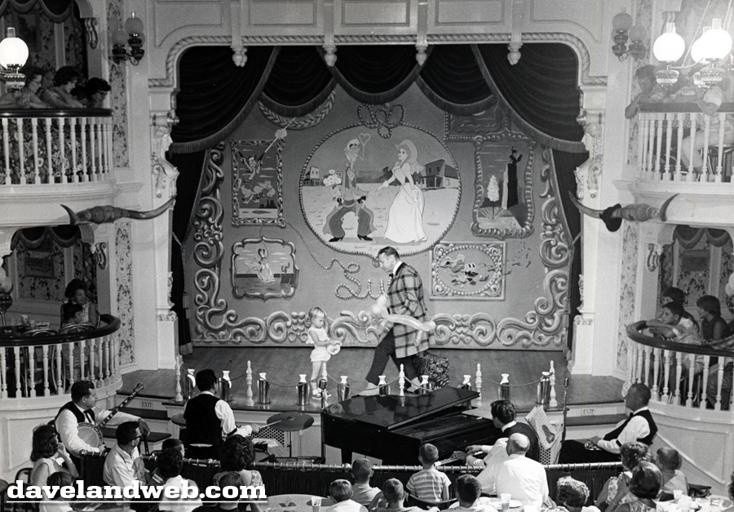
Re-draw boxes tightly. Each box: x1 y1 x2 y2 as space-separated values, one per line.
0 26 30 90
610 11 647 63
108 9 146 67
652 16 733 90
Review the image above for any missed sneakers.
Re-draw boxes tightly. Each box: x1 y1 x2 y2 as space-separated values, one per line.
312 389 332 401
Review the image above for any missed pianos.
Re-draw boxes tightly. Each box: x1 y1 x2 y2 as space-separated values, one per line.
321 385 502 465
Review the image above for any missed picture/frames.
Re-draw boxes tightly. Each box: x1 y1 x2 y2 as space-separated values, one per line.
230 236 298 304
429 238 507 300
442 105 513 143
228 135 286 228
470 130 538 240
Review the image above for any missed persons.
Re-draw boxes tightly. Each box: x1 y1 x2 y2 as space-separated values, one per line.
374 139 427 243
234 148 277 209
623 65 734 411
358 247 436 396
0 66 111 182
306 307 342 401
323 139 378 242
59 279 97 378
31 371 734 511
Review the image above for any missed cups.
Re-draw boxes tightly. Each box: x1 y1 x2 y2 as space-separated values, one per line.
21 314 36 328
671 489 698 512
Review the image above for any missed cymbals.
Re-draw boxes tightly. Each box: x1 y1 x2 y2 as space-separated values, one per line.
171 414 187 426
265 411 314 432
